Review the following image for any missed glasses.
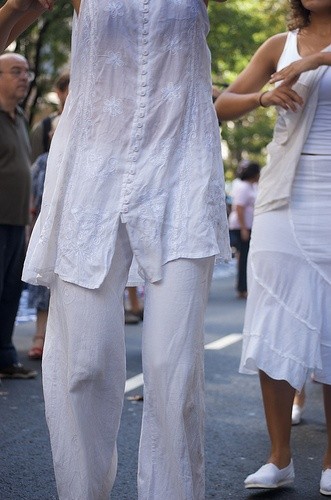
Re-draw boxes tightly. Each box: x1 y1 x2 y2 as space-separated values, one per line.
0 70 34 81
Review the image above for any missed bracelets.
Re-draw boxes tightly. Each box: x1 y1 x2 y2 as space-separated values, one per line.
259 91 269 108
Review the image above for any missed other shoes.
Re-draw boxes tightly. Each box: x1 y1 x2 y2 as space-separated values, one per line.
244 458 296 488
319 469 331 496
291 404 304 425
124 308 144 324
29 336 45 359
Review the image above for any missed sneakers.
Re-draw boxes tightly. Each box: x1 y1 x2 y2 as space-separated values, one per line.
0 362 38 378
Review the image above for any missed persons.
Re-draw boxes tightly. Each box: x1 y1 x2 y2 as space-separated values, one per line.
0 0 304 500
213 0 331 496
